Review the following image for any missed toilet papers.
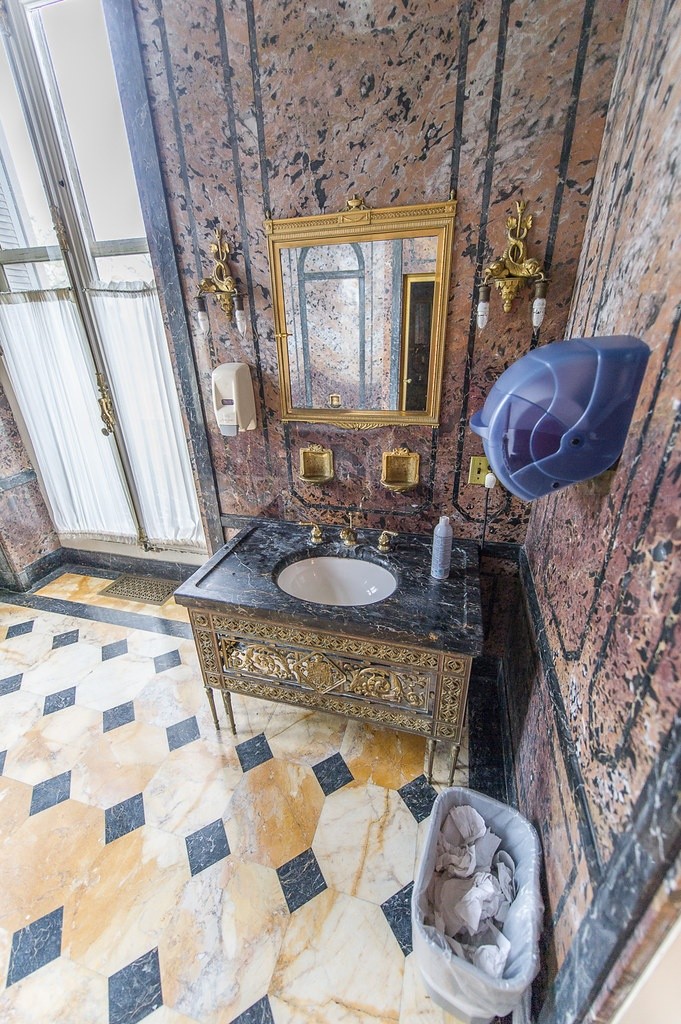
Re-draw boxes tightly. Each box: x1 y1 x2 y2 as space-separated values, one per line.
507 385 589 458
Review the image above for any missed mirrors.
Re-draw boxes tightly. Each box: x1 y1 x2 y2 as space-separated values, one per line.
263 189 458 431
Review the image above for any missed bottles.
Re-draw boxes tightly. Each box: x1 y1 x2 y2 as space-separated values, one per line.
431 514 453 579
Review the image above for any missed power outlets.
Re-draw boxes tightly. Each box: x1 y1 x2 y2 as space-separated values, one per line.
467 456 500 487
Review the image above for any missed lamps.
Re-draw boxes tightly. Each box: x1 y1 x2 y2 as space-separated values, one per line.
192 225 249 342
475 200 551 336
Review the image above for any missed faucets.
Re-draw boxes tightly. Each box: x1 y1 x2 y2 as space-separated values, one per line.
340 529 357 545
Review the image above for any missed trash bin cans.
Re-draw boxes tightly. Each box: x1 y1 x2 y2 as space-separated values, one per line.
410 786 543 1023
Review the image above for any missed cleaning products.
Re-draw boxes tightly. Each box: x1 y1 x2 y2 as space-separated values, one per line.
431 516 453 579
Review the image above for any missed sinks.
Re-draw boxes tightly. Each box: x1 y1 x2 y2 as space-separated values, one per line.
271 546 399 606
